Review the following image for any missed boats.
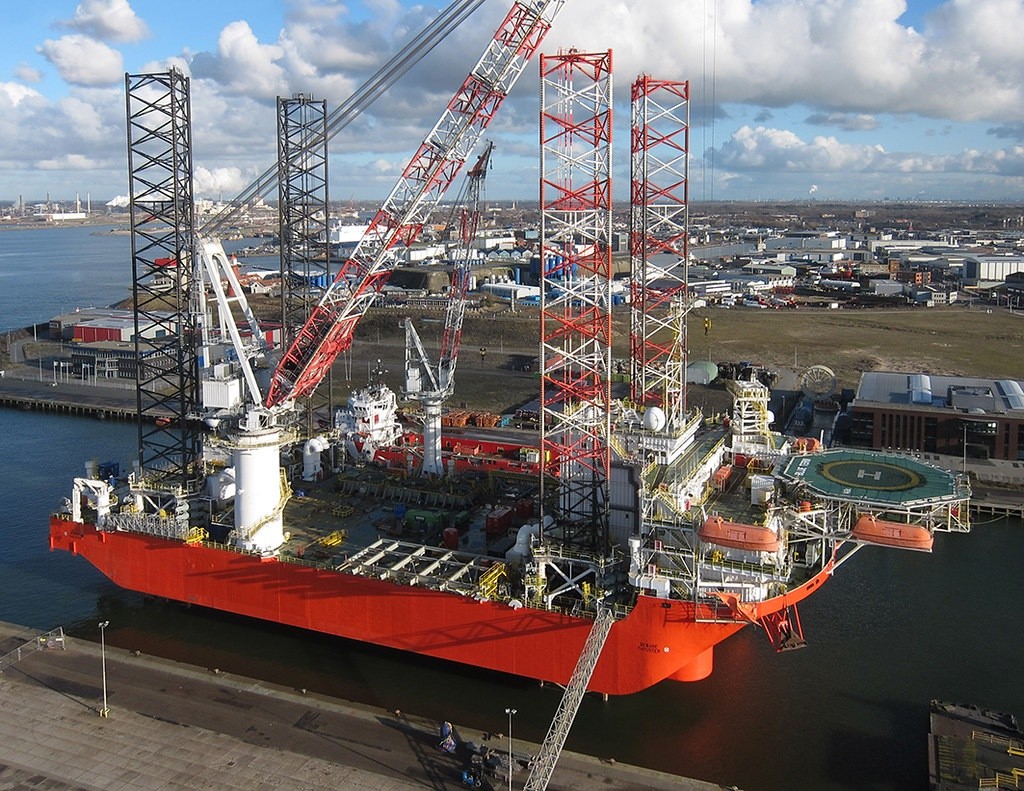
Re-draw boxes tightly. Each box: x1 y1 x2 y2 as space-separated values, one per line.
43 0 868 697
850 511 935 553
697 514 780 554
